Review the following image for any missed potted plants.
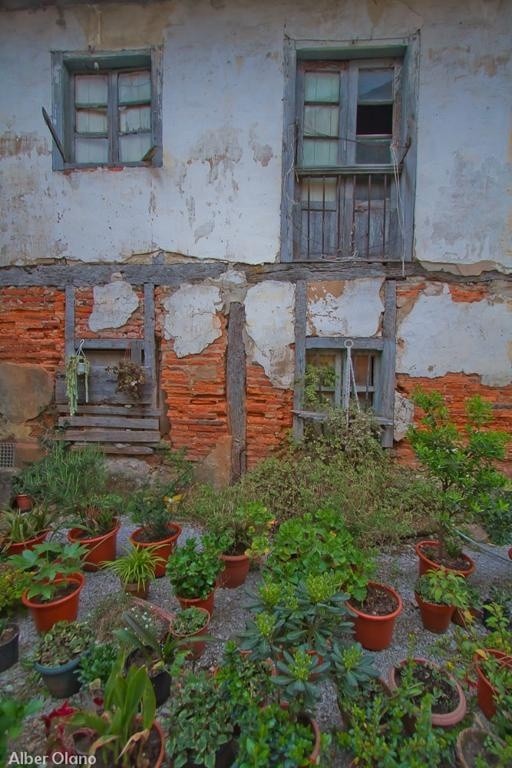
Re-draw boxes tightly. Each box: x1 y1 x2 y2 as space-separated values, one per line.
0 384 512 768
